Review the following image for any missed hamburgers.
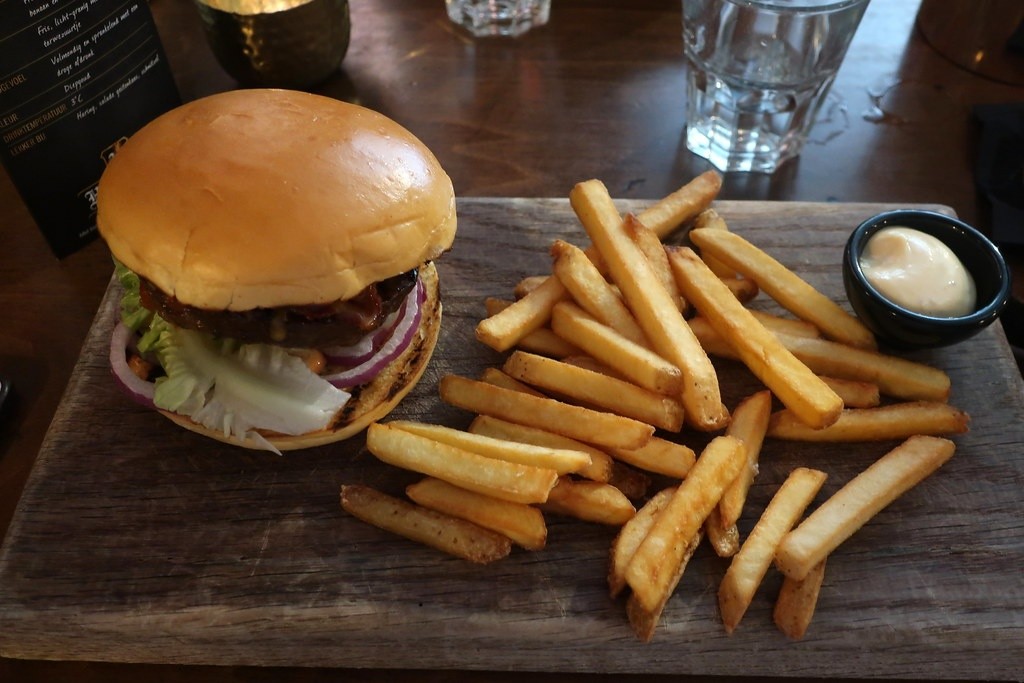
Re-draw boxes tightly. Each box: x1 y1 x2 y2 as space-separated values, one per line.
97 89 457 453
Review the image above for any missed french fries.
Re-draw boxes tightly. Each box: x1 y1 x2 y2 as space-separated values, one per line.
340 168 969 642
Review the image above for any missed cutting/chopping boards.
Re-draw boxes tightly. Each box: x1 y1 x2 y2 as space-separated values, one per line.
0 198 1024 683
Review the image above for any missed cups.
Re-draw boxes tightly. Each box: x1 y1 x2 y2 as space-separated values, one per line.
193 0 350 89
444 0 549 38
682 0 873 175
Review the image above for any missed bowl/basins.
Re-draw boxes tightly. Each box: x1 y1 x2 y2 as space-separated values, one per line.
841 208 1011 351
